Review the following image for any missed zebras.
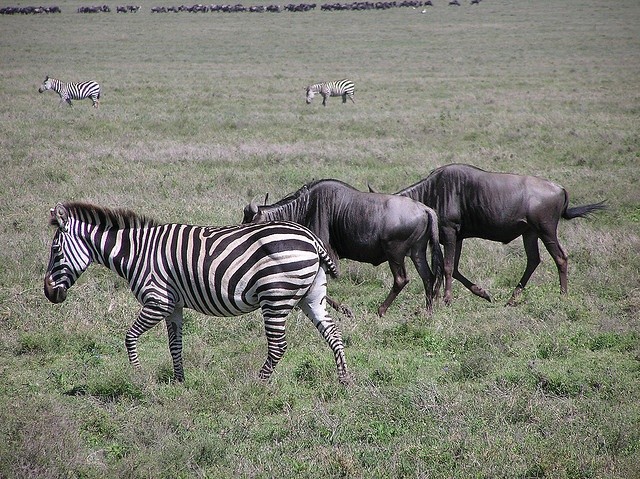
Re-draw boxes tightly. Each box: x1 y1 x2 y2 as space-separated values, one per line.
302 79 355 107
43 201 351 388
37 76 101 109
